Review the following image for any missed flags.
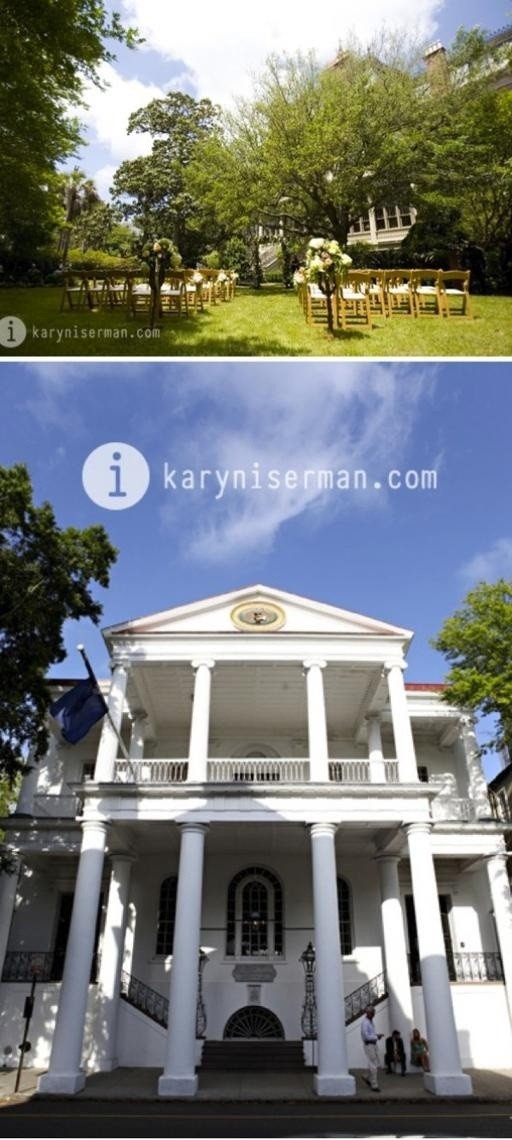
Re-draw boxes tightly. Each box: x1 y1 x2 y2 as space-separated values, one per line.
49 675 110 747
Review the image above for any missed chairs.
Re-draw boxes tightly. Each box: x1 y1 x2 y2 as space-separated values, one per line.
54 266 240 323
296 266 473 331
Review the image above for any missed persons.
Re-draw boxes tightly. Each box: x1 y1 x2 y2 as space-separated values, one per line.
360 1006 385 1093
383 1030 408 1078
410 1028 431 1072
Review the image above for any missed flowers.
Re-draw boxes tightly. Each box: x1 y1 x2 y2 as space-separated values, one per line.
140 237 182 271
294 237 354 286
